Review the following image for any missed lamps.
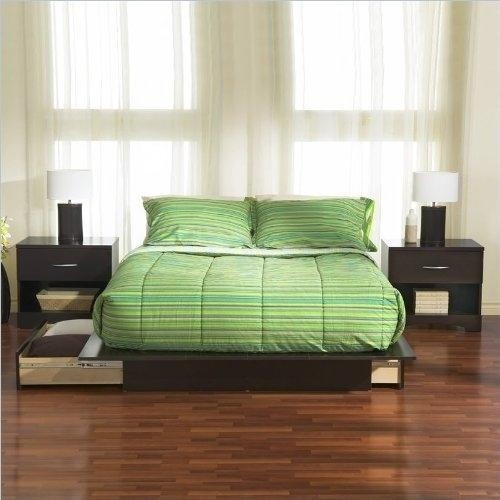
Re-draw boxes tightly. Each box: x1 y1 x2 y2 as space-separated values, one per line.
413 170 459 247
47 166 94 245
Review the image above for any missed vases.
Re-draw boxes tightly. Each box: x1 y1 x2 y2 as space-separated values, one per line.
1 261 11 324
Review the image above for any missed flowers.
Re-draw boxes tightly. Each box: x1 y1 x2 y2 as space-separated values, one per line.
0 216 16 263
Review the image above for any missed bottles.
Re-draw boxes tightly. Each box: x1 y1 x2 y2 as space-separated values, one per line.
406 210 418 224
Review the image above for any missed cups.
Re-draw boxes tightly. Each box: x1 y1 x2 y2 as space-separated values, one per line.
405 225 417 244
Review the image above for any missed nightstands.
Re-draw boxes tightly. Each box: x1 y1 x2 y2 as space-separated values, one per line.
16 236 119 330
381 238 484 332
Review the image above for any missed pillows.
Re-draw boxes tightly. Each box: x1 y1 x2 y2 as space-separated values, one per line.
250 197 378 252
143 198 251 249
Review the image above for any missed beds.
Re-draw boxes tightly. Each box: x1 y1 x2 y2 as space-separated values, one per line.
14 194 416 393
